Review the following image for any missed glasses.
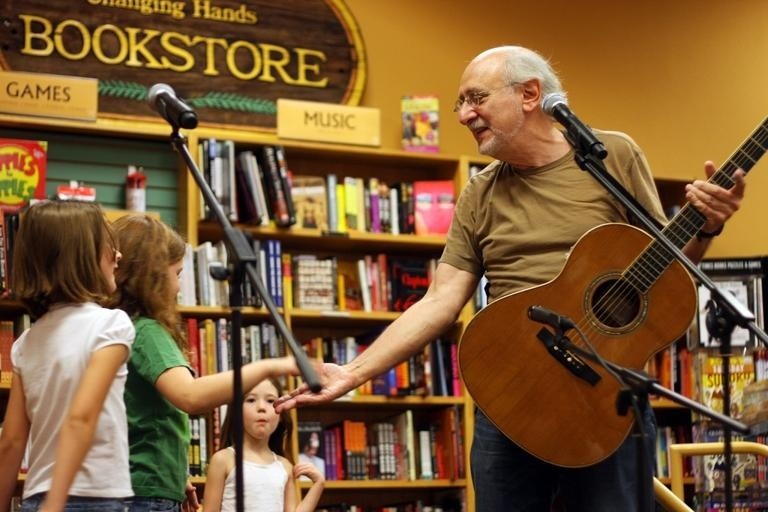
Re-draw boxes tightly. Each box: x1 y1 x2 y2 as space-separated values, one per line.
453 86 514 110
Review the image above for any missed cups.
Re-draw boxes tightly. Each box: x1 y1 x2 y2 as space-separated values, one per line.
125 174 147 214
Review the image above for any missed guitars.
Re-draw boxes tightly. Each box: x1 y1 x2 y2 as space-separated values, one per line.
458 115 767 468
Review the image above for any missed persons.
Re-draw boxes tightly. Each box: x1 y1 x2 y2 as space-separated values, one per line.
273 46 746 512
1 199 135 512
106 214 328 512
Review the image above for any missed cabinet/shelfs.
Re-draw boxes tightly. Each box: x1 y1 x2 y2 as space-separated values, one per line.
643 255 764 510
654 177 694 221
193 120 477 511
464 154 496 174
0 303 32 480
0 114 192 302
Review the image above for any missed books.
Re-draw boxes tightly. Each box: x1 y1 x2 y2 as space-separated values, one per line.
644 204 768 512
1 137 48 512
397 94 440 152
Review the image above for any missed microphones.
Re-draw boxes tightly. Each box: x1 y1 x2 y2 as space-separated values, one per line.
527 305 577 333
542 92 607 160
150 82 197 129
